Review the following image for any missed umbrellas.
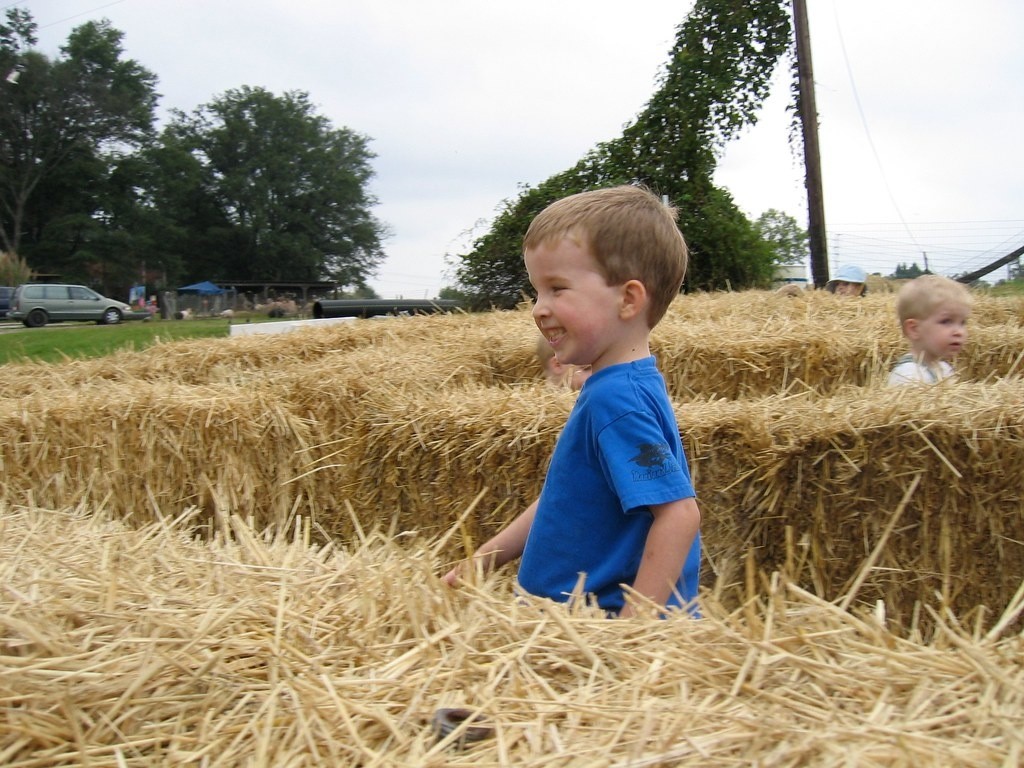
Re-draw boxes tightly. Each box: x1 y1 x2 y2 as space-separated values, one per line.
178 281 230 314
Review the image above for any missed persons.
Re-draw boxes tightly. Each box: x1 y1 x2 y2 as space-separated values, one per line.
440 184 701 621
133 296 317 321
882 274 971 389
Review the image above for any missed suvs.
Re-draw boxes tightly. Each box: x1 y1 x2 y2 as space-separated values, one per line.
4 284 132 328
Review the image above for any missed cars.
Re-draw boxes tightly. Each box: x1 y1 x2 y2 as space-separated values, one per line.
0 287 17 322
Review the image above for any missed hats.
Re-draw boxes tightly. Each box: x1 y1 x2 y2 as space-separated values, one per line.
828 265 866 283
